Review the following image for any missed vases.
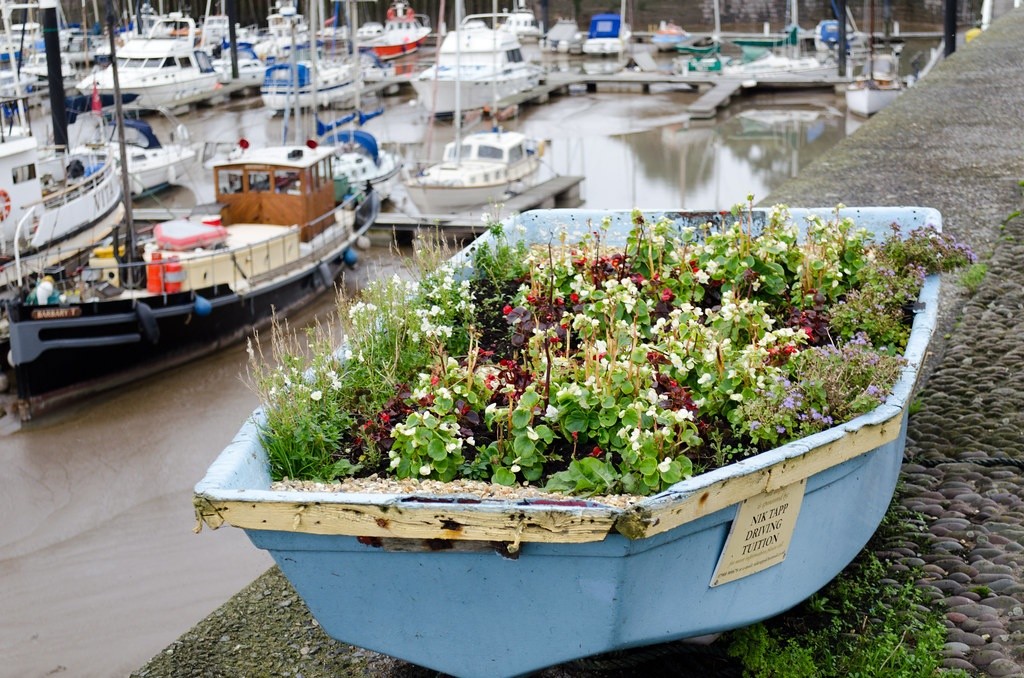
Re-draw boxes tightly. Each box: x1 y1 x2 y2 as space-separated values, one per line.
194 203 946 678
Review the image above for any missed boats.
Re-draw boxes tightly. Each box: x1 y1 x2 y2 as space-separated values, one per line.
0 0 945 428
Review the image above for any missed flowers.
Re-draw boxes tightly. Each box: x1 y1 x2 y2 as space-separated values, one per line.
239 186 977 493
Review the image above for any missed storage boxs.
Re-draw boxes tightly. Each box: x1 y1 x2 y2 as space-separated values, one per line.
89 253 123 289
